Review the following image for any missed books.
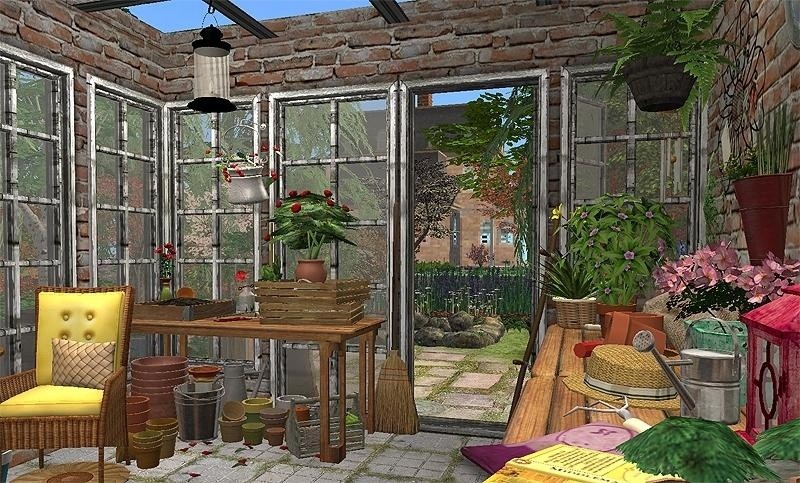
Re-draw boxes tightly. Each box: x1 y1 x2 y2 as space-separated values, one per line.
480 442 686 483
462 419 640 477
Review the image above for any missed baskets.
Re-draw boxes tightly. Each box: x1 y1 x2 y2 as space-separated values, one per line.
286 392 366 458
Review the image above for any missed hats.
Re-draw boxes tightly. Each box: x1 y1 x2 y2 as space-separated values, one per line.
563 344 691 411
